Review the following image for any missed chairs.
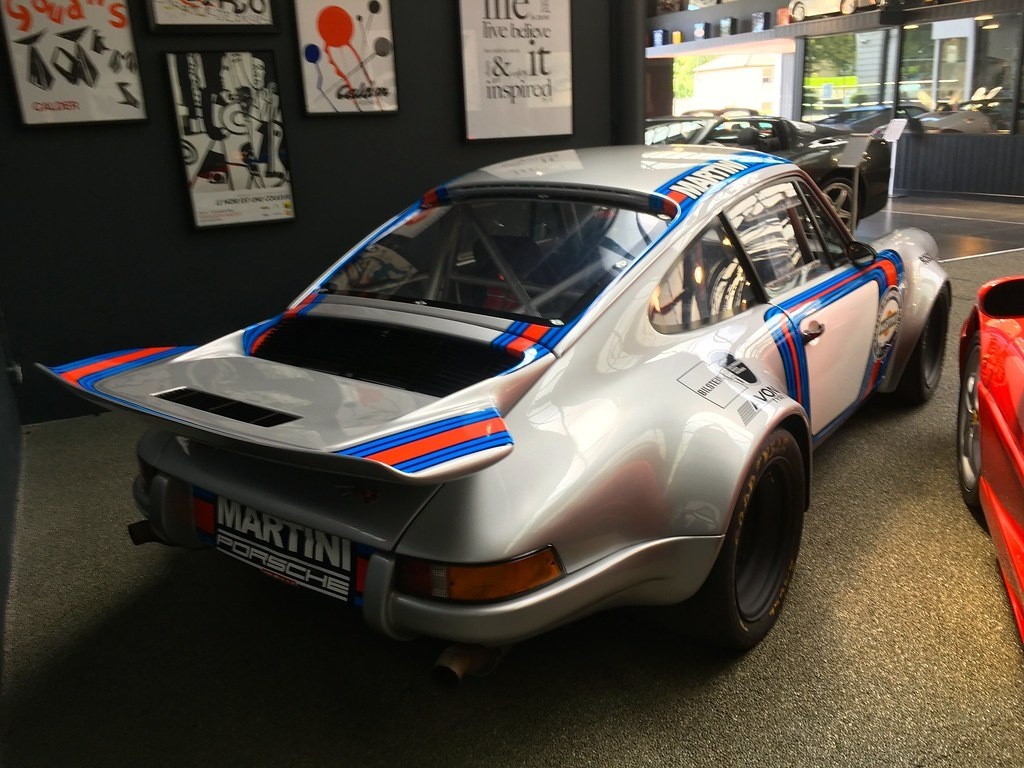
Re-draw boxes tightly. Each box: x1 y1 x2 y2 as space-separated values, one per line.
734 127 768 153
471 234 552 286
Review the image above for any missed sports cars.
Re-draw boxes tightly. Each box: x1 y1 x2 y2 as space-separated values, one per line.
641 106 893 241
810 98 1023 134
28 143 957 656
956 272 1024 640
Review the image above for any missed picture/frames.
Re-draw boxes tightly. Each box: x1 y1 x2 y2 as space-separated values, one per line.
0 0 153 128
140 0 283 37
289 0 403 118
455 1 576 142
160 48 300 231
